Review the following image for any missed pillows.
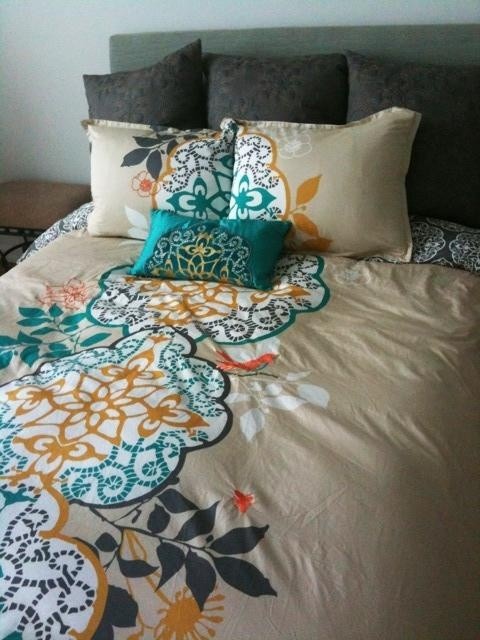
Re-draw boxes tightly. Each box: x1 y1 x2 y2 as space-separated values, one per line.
84 39 202 128
225 107 425 267
0 178 91 275
200 53 343 128
346 47 479 232
128 209 293 292
83 119 235 246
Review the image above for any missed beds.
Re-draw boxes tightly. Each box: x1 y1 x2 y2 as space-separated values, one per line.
2 23 479 638
